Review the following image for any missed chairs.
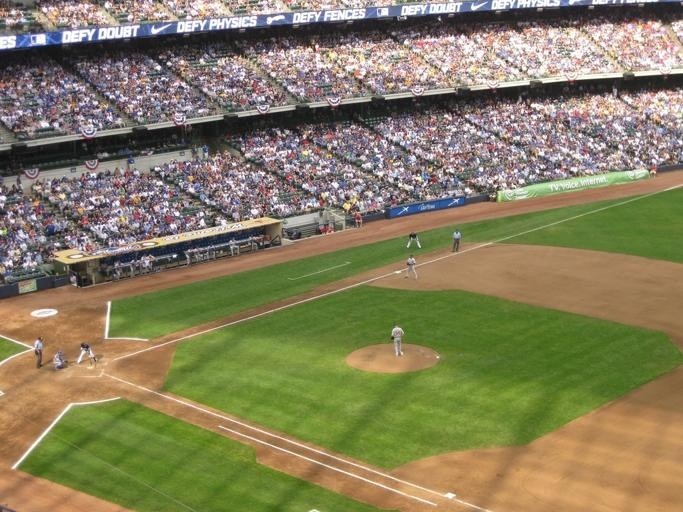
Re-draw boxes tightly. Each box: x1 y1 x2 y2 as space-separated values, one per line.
2 2 682 281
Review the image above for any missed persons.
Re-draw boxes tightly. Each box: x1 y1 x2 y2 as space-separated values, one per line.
76 342 99 365
390 323 407 357
53 350 69 369
34 336 46 368
1 0 683 288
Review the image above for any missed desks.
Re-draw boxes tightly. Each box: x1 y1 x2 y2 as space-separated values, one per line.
110 234 264 281
